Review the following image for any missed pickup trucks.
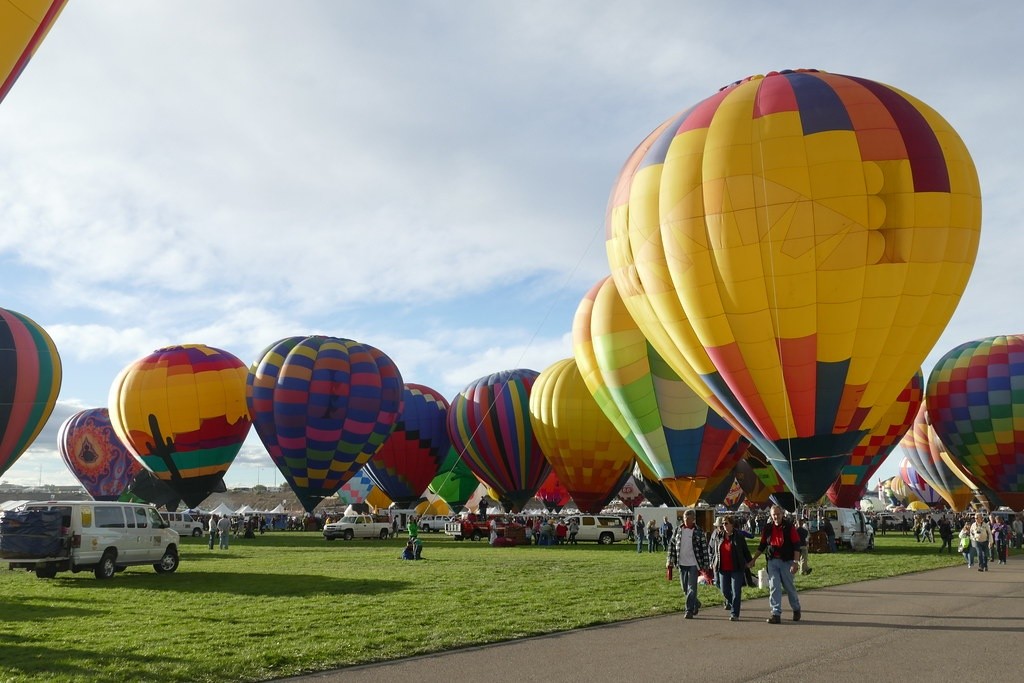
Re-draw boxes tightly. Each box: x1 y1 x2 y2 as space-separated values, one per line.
552 516 628 545
420 515 452 533
863 513 913 530
324 515 393 541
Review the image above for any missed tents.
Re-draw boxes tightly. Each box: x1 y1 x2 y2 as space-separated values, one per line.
181 505 209 515
210 502 235 516
234 503 288 516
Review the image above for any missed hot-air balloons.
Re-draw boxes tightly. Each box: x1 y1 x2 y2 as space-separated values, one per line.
246 335 404 531
883 312 1024 515
57 343 252 516
0 308 63 481
336 67 983 517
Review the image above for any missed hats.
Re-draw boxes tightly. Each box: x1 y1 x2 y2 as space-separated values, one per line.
713 516 724 525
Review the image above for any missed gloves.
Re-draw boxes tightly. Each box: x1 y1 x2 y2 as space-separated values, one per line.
667 567 672 580
701 569 713 585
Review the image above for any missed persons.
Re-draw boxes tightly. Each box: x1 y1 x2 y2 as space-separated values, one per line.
667 510 709 619
1012 513 1024 549
709 516 755 621
752 505 801 624
938 517 953 553
478 495 488 520
626 517 634 540
635 514 645 553
801 516 823 551
660 515 673 551
494 512 579 546
959 521 973 567
715 512 796 535
646 519 659 553
821 516 839 553
408 514 421 550
402 536 422 560
955 512 1012 571
865 510 936 542
192 513 294 549
390 513 400 538
295 511 340 532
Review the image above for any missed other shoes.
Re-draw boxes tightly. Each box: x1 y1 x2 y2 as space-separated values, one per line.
793 605 801 620
694 601 702 615
767 615 782 624
724 601 731 609
684 610 692 619
729 615 740 621
977 567 984 572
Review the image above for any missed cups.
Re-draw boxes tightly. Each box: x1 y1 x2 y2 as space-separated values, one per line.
667 565 673 581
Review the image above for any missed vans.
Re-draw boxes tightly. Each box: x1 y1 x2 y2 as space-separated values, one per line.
152 512 203 537
804 506 874 551
22 501 179 581
967 512 989 523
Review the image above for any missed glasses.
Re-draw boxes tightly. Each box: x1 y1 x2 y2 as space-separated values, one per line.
722 522 729 525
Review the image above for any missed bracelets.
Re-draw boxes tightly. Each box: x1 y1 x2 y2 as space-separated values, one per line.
793 560 798 564
